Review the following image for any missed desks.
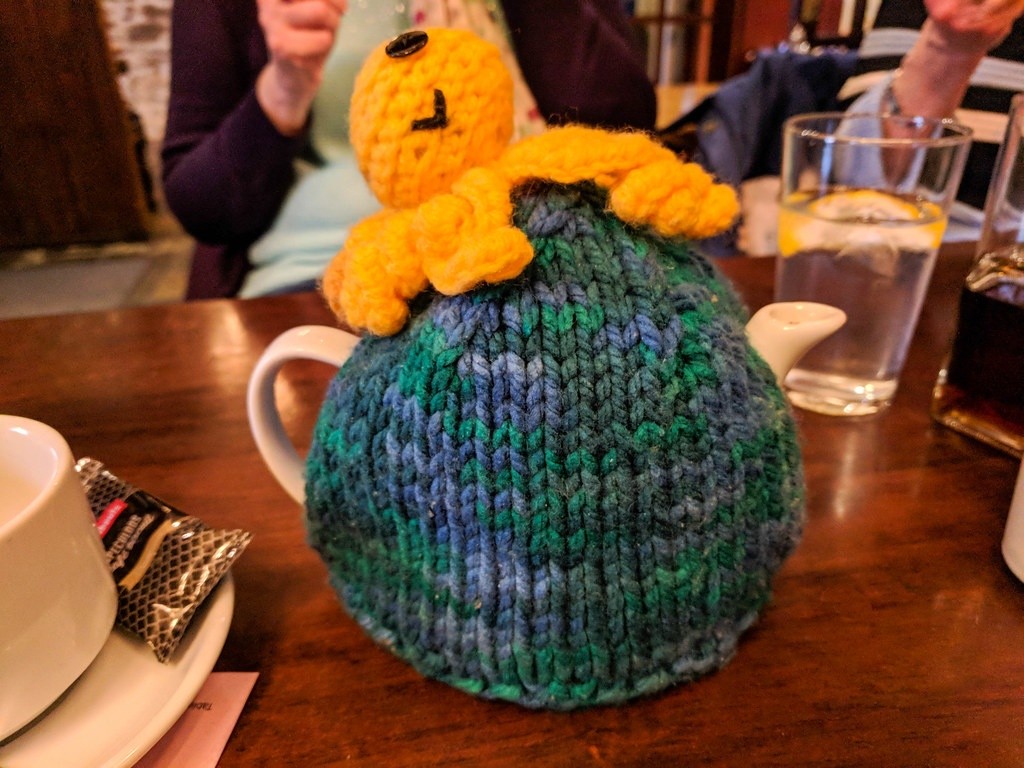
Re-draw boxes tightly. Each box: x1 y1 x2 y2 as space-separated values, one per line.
1 226 1024 767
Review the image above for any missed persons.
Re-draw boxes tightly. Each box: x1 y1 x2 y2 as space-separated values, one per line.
819 0 1024 212
161 0 657 304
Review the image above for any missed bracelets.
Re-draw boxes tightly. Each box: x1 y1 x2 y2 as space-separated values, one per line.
882 79 915 128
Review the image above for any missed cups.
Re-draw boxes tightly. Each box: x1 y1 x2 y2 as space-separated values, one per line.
776 112 972 415
0 415 117 741
932 94 1024 459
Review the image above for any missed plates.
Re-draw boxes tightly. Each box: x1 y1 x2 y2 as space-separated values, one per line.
0 568 235 768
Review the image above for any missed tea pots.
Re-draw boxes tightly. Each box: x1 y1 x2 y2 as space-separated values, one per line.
245 24 846 710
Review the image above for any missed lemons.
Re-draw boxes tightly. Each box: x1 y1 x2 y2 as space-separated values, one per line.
796 191 938 253
